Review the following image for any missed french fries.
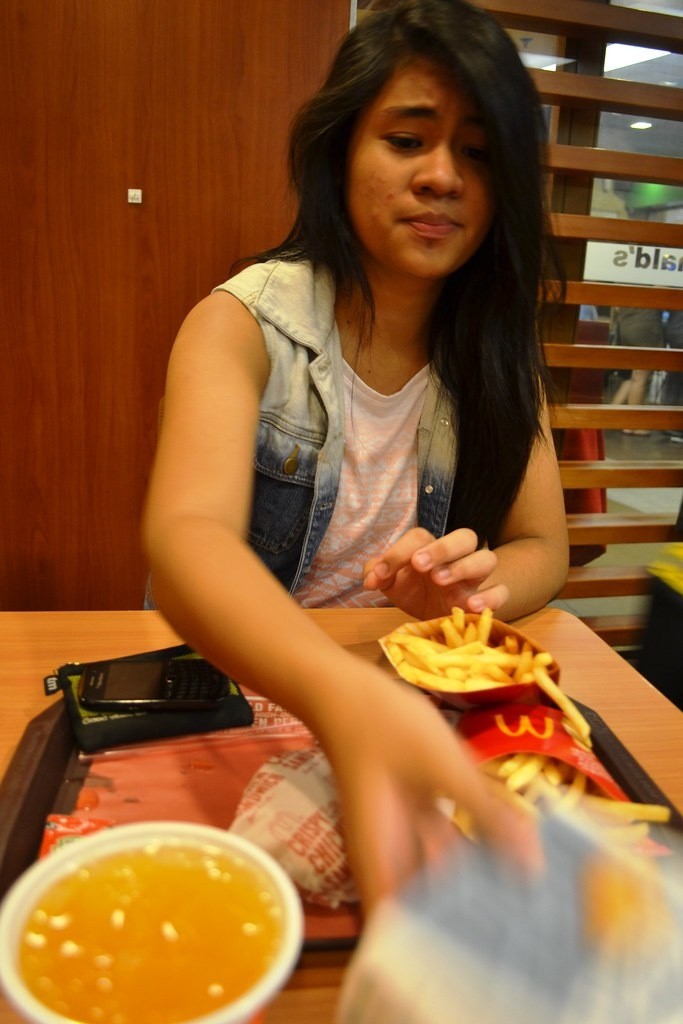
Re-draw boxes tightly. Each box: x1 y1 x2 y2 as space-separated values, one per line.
385 605 591 744
453 755 671 845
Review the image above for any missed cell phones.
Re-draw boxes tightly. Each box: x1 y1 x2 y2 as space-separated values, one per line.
78 659 230 712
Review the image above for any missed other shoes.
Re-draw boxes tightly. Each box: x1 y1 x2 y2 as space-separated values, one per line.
622 429 650 436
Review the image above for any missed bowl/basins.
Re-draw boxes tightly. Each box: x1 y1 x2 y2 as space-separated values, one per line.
0 819 303 1024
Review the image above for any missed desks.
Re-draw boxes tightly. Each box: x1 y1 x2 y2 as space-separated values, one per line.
0 604 683 1024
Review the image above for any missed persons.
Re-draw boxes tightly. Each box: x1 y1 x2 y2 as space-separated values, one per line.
142 1 571 911
609 303 683 441
